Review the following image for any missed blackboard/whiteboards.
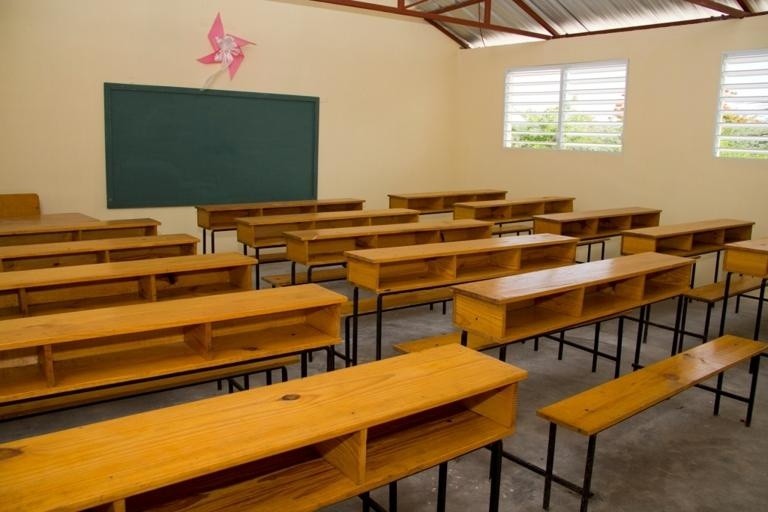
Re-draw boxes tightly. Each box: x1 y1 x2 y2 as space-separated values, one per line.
105 83 320 209
